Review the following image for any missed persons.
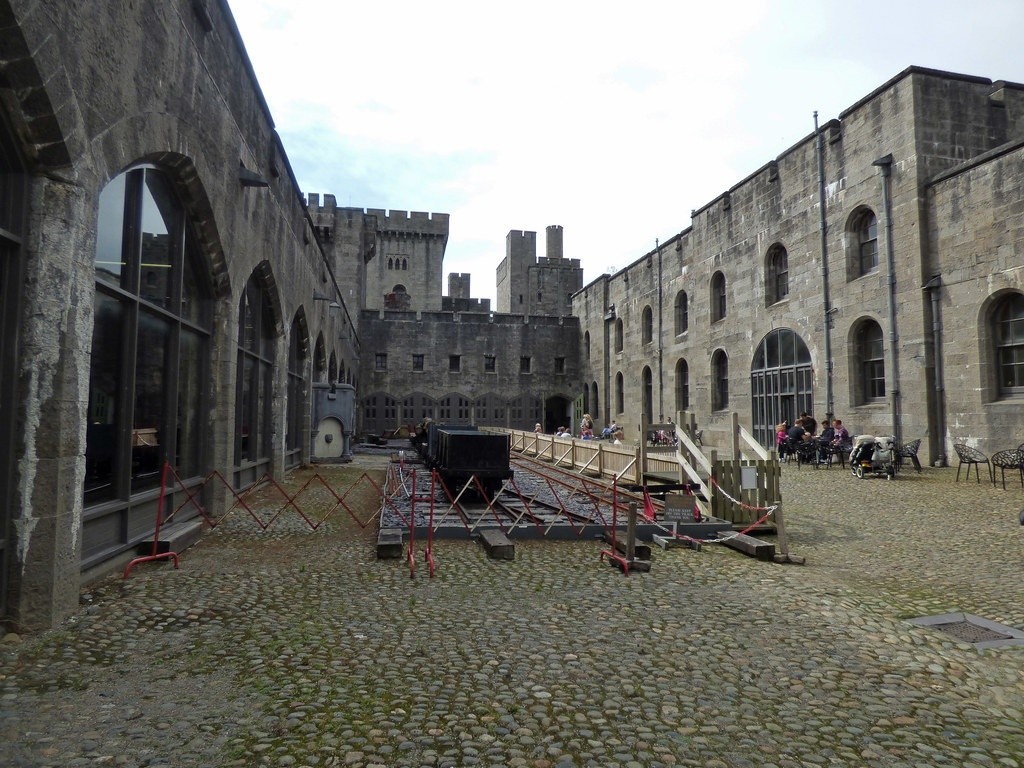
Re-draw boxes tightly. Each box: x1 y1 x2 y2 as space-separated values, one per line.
800 412 816 436
561 428 571 437
815 421 835 464
652 416 674 444
830 420 850 450
580 414 593 440
534 423 542 433
600 421 617 439
788 419 811 464
556 426 565 436
776 418 788 462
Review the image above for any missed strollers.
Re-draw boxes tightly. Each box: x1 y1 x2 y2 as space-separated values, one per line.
849 434 896 479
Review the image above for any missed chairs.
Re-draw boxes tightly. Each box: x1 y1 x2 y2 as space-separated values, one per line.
954 444 992 483
646 430 704 447
1017 443 1024 488
990 449 1024 490
782 436 924 474
